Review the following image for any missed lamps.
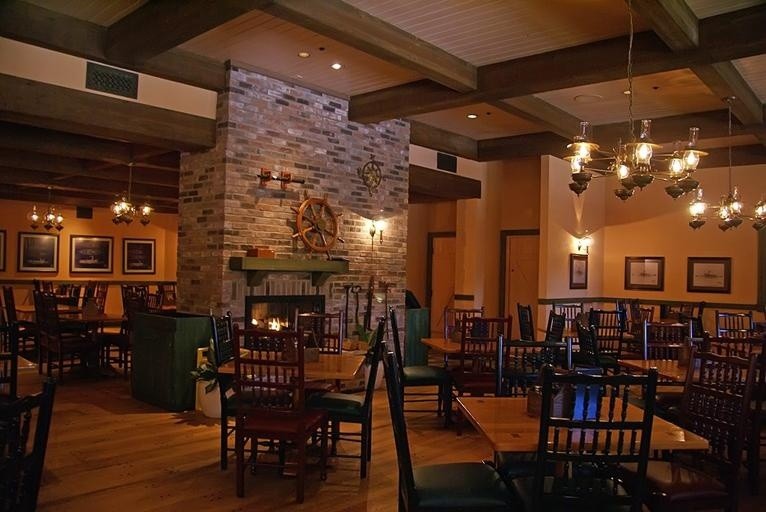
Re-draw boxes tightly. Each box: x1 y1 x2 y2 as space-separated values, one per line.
109 164 156 226
26 187 64 231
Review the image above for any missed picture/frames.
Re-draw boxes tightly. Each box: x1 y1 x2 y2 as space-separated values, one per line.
1 229 8 272
622 254 665 292
686 256 734 295
569 253 589 291
122 238 156 274
16 230 59 274
69 235 114 273
